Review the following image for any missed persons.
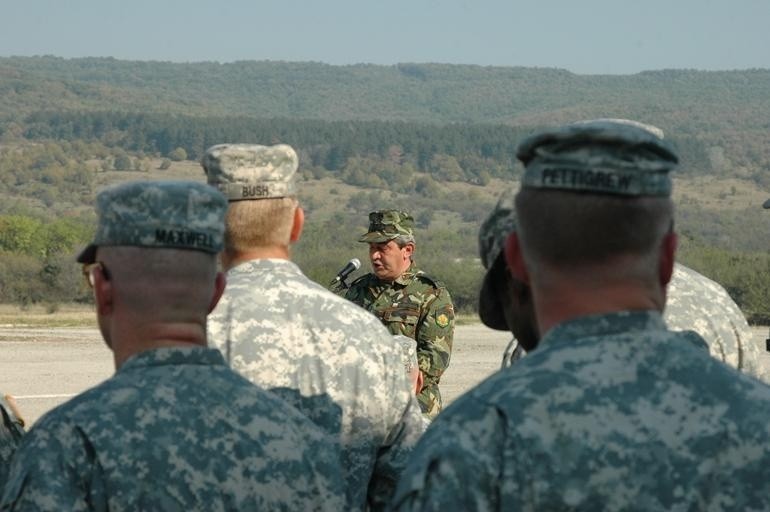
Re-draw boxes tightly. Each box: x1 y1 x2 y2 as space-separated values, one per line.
0 400 25 478
345 209 455 420
1 182 353 512
388 118 770 512
201 143 430 512
477 181 767 382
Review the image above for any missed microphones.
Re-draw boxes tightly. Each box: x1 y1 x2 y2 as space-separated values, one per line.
331 258 361 284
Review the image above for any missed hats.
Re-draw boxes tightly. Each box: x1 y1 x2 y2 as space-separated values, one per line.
77 182 227 264
514 119 679 196
478 182 527 330
205 142 298 202
358 208 416 243
